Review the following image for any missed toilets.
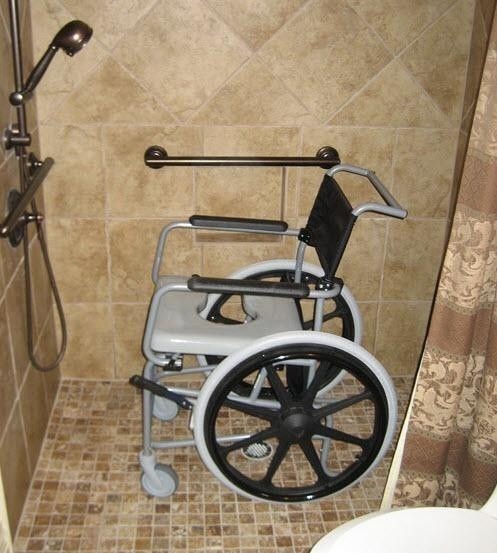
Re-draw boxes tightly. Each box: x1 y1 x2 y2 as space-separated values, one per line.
307 481 497 553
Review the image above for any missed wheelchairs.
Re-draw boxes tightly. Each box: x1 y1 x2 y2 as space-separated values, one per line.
128 164 408 505
